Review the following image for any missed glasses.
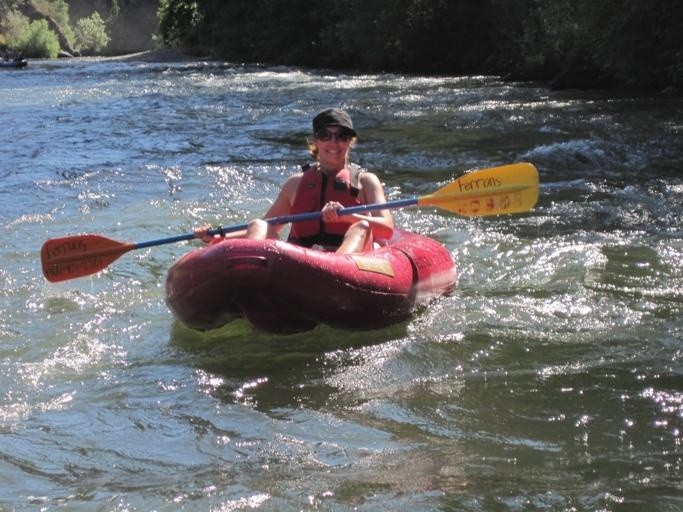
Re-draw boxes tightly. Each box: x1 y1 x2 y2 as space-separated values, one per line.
315 129 349 142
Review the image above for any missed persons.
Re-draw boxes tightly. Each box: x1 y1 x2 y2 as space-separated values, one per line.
194 108 395 255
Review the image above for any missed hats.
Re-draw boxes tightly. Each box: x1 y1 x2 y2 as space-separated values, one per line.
312 107 358 137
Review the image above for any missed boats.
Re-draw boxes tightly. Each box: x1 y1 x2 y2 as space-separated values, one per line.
164 227 460 334
0 58 28 67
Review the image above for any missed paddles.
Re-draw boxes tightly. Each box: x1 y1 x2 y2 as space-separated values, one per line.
40 162 539 283
